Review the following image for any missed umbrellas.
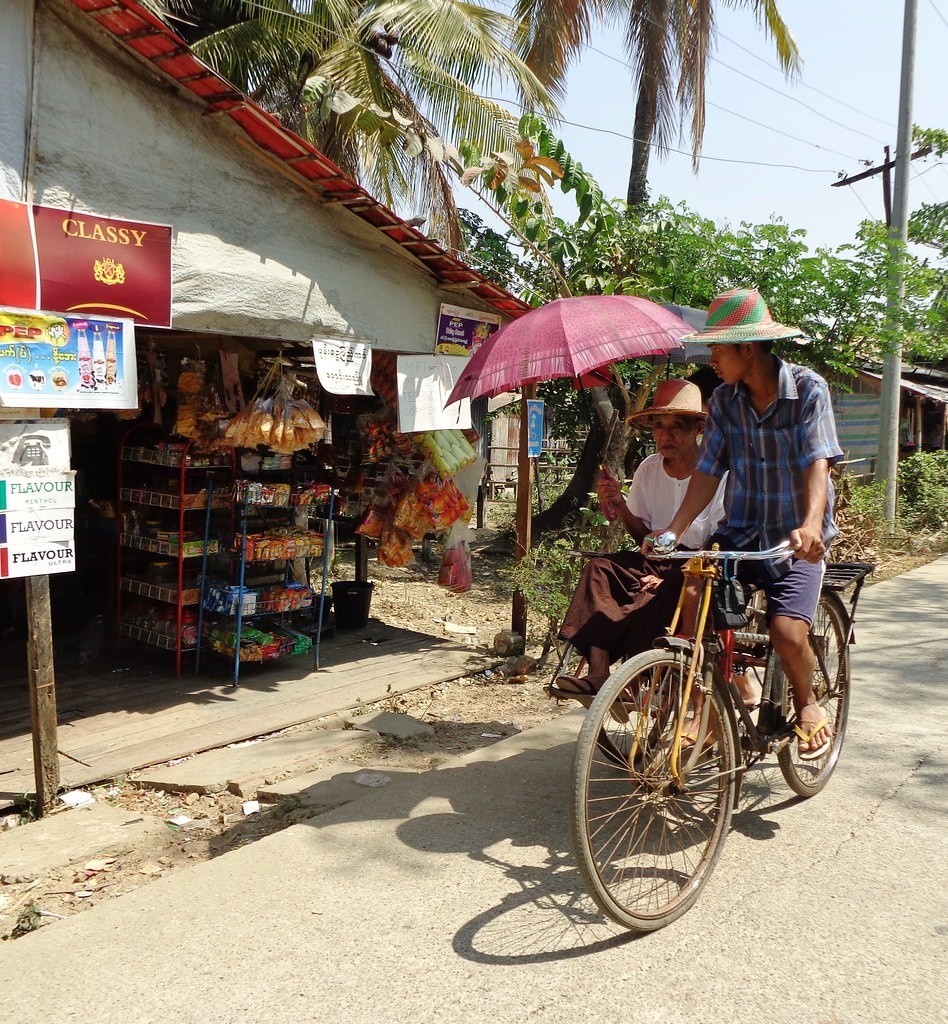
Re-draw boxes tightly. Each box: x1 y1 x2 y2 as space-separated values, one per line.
442 295 712 522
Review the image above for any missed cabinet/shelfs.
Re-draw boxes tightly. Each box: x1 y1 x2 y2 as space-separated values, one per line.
114 422 336 689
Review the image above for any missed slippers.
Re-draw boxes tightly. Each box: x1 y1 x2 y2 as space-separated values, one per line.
659 733 714 753
793 707 830 761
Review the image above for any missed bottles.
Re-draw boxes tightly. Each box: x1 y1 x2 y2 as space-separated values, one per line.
72 320 120 390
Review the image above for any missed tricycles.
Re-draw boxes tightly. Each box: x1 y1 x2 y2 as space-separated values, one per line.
542 531 874 933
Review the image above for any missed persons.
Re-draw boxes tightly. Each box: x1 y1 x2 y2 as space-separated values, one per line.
557 366 757 723
641 288 844 761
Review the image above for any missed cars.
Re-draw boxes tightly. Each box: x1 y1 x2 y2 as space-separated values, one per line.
574 431 589 442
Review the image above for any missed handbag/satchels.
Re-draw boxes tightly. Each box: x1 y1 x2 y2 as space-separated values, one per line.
437 522 476 593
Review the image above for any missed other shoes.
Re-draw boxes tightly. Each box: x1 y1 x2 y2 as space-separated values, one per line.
622 688 633 713
556 676 592 710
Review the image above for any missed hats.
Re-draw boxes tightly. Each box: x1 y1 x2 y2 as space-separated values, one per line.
679 290 804 343
626 379 708 432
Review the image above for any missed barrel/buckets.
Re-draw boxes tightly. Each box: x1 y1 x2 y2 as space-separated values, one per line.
330 581 376 630
